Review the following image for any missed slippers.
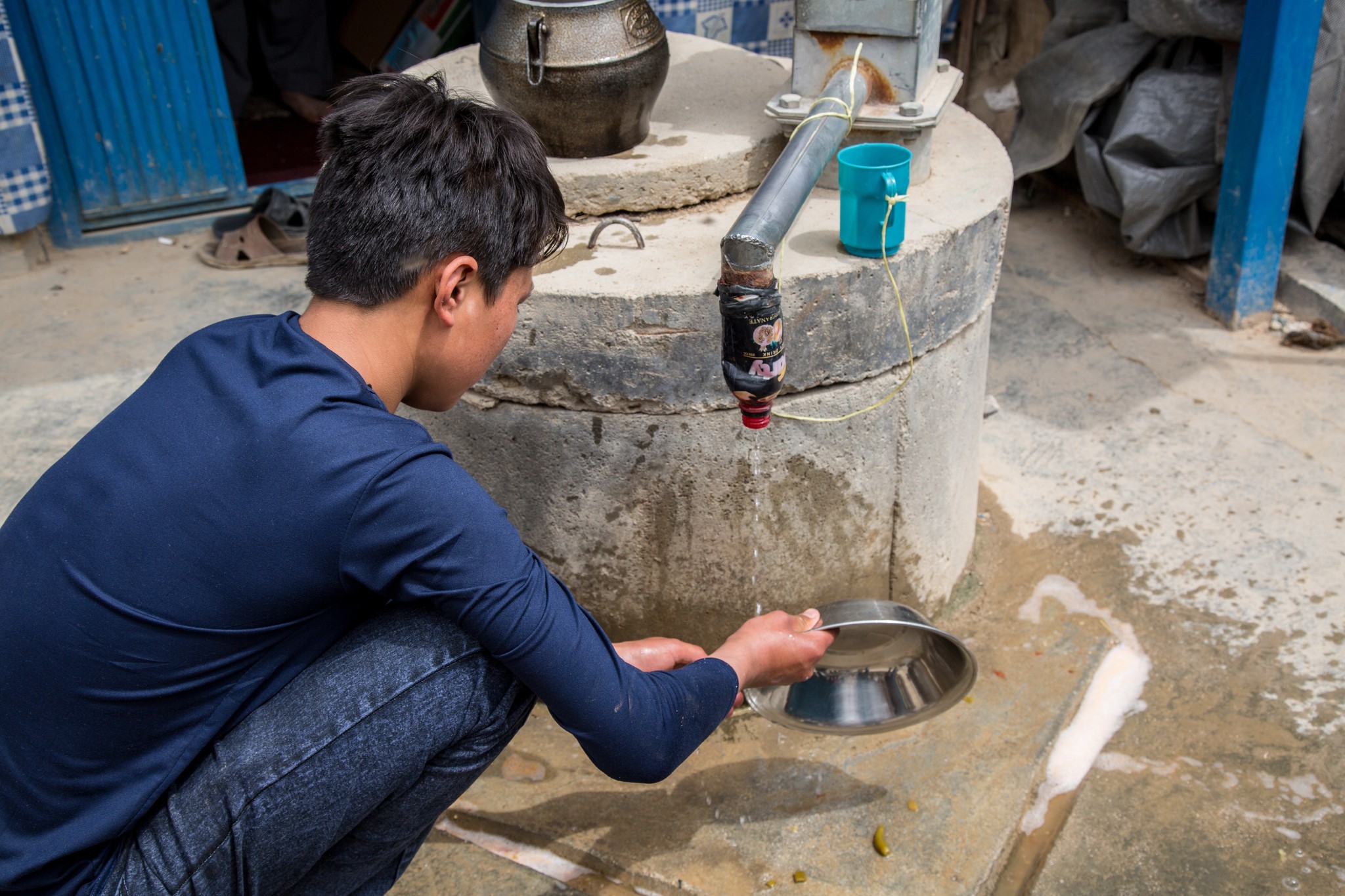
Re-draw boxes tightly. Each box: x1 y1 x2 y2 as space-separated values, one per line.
212 187 311 239
197 214 311 270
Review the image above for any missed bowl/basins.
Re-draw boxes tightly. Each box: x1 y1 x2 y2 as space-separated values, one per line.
744 600 977 735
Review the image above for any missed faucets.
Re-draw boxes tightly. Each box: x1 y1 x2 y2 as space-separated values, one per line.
714 61 873 428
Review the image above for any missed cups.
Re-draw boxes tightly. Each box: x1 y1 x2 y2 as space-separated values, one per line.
838 141 913 255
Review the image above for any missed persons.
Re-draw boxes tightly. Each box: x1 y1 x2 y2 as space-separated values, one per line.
0 74 841 896
